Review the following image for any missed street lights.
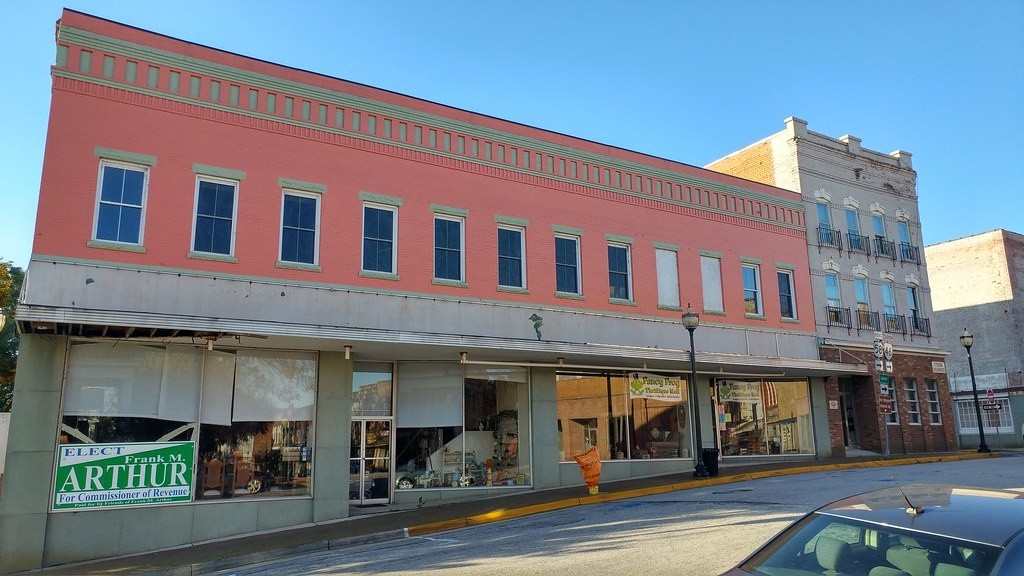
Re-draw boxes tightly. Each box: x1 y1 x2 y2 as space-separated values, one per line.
681 303 713 480
959 327 991 453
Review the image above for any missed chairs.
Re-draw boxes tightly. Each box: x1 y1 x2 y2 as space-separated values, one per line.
885 536 942 576
933 546 980 576
814 537 852 576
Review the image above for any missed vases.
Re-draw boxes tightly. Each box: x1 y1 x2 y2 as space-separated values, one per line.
650 428 660 440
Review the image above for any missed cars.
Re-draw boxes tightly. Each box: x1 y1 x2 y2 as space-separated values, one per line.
720 486 1024 576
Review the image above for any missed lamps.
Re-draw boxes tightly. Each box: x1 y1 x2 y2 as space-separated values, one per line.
208 340 213 350
345 347 350 359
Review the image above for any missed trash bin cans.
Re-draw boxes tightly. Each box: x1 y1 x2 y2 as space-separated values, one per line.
700 447 719 477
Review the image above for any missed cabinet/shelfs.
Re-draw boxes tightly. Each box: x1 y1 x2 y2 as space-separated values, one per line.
443 450 476 483
648 442 679 458
